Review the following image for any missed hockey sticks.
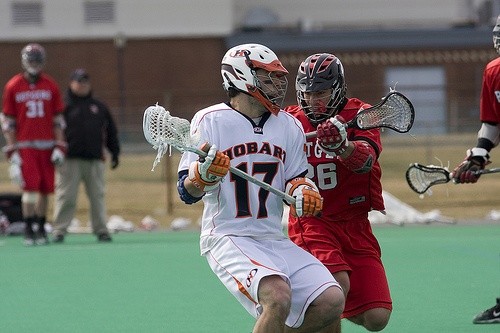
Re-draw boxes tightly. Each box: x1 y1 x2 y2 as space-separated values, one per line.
142 104 322 217
405 163 500 193
306 91 416 138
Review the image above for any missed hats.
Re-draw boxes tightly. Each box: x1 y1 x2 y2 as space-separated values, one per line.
72 70 89 81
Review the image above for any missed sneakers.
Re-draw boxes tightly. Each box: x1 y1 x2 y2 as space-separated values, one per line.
473 298 500 323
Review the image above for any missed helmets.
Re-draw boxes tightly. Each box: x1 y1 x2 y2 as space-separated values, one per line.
221 43 289 117
295 53 344 125
492 15 500 53
21 44 45 74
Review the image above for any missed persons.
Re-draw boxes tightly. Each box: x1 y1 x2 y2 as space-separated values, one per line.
280 54 392 333
177 44 345 333
448 18 500 323
0 42 65 246
51 70 121 243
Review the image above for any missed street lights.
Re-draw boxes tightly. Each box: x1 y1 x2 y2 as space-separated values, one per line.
113 35 126 132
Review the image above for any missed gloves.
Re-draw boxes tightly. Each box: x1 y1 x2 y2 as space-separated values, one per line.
7 150 22 166
110 154 119 167
51 146 65 166
452 148 491 184
316 114 349 157
188 142 230 192
284 178 324 217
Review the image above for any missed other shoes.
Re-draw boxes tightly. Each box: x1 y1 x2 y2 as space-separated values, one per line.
100 234 111 240
54 235 62 241
